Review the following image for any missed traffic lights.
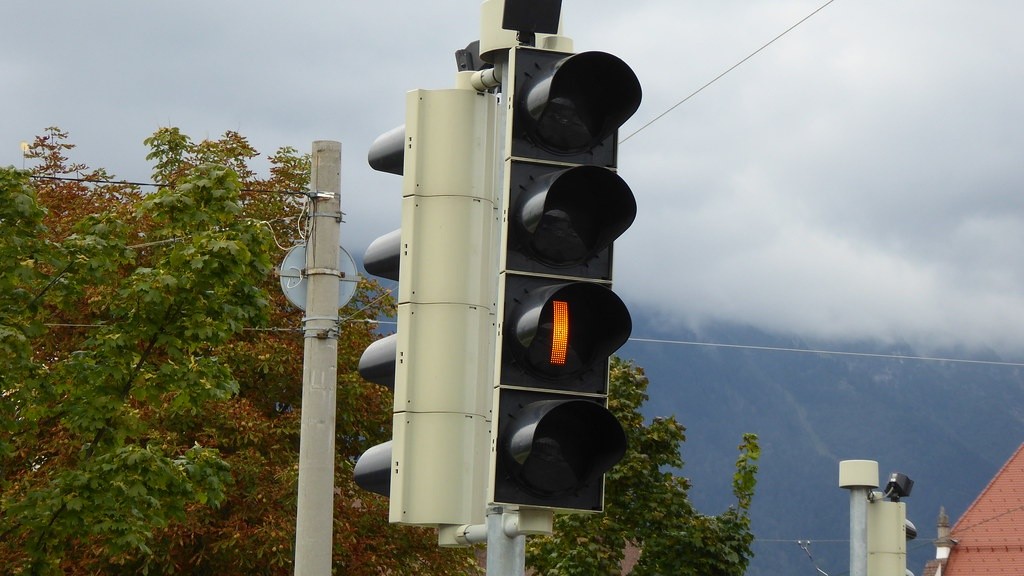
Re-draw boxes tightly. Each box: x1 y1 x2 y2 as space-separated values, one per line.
351 86 501 528
473 43 643 517
